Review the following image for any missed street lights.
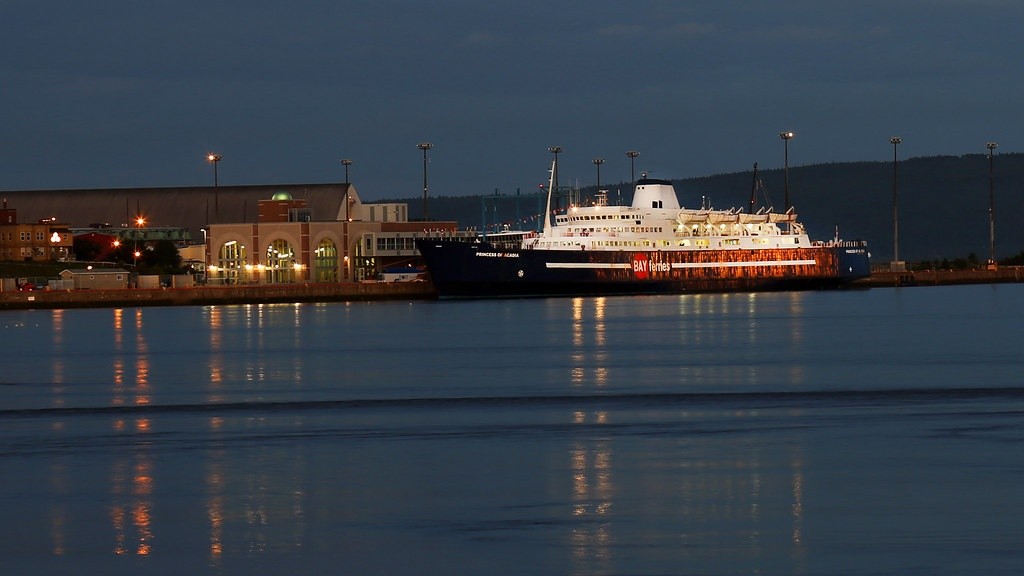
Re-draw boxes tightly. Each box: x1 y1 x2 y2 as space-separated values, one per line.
341 159 353 220
625 151 640 202
591 158 605 196
208 154 222 223
547 146 562 214
778 131 793 214
417 142 434 223
890 136 901 262
985 142 998 263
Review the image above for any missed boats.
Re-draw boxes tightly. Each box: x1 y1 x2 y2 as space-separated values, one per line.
412 176 876 295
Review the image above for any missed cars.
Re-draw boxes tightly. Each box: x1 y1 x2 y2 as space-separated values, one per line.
32 283 44 290
